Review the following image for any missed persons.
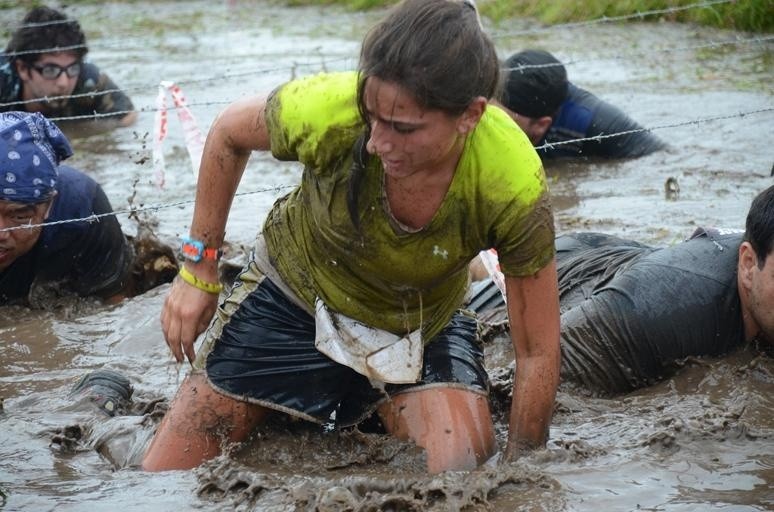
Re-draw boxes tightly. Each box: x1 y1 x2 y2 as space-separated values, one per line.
440 179 773 404
474 48 682 174
53 1 564 481
0 109 142 315
0 4 137 138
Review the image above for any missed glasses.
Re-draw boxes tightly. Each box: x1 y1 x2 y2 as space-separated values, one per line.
29 60 83 80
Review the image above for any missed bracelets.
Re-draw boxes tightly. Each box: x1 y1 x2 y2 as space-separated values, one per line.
176 240 224 263
177 265 224 294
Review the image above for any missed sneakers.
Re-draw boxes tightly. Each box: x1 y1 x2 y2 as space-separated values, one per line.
54 371 135 418
